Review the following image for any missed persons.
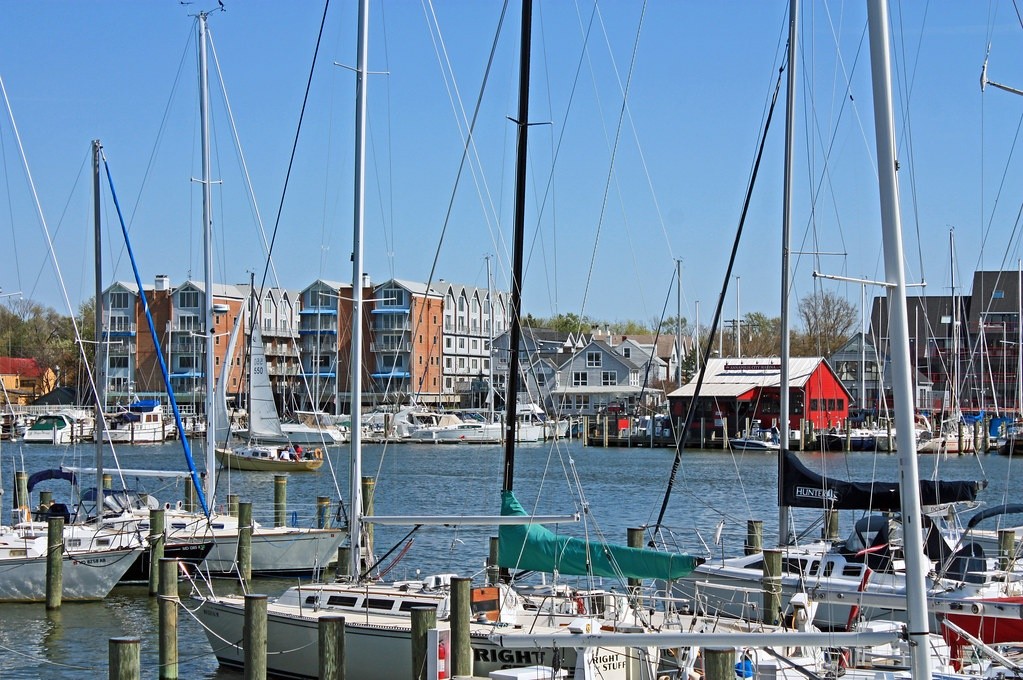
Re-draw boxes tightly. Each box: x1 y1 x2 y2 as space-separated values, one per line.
772 424 780 445
279 445 312 461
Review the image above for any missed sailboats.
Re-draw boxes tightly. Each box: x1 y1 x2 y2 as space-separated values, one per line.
2 0 1023 680
